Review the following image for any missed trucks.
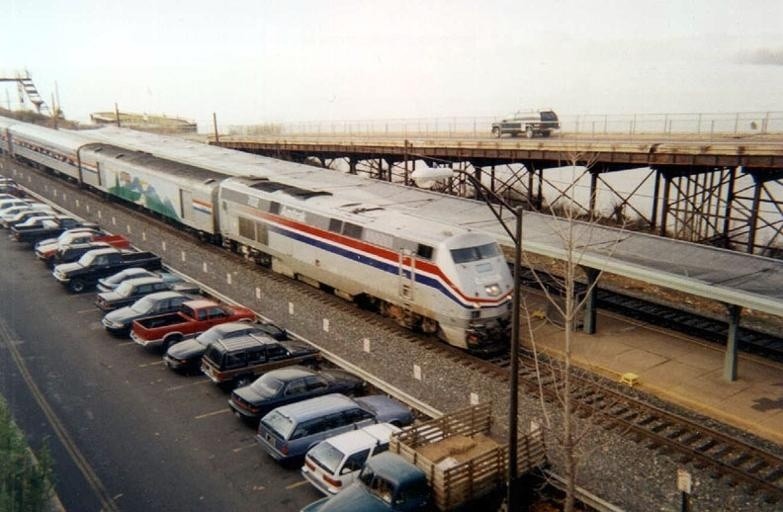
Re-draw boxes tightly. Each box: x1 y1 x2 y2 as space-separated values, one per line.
294 399 552 512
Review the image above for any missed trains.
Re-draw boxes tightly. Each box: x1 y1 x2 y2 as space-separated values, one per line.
0 107 518 354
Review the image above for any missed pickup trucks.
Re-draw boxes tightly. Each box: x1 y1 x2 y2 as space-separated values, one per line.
128 298 259 352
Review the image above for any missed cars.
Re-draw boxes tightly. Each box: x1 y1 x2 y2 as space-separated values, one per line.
1 172 209 336
166 322 447 497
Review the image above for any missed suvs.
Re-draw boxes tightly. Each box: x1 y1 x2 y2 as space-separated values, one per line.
490 109 561 139
